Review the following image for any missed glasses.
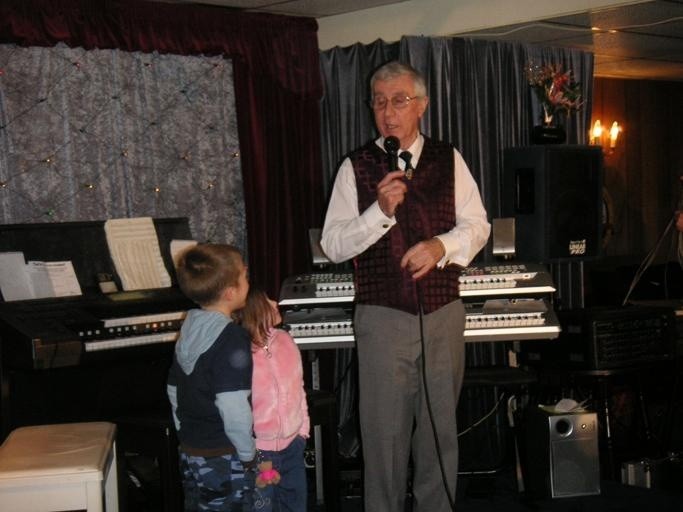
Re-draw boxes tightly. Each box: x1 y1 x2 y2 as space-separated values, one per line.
370 96 418 109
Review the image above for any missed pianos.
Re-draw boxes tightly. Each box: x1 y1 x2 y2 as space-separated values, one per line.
278 260 559 306
1 215 196 511
278 297 566 351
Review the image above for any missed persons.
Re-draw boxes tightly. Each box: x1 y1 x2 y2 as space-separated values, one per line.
319 61 492 511
166 246 257 511
230 285 310 512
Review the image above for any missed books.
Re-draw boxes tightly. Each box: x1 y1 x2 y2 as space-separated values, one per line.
0 249 80 301
105 217 172 293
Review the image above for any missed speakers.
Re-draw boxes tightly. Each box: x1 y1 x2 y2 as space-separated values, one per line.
512 410 602 499
502 145 604 264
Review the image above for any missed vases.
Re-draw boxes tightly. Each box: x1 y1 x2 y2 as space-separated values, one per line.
534 125 567 142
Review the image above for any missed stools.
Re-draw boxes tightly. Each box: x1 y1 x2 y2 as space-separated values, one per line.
0 421 119 510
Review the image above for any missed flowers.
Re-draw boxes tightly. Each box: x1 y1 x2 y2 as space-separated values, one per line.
526 60 586 125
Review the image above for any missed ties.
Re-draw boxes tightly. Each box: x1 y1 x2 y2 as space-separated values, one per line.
399 151 414 180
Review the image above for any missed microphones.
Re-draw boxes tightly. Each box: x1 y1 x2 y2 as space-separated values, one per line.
383 135 401 180
